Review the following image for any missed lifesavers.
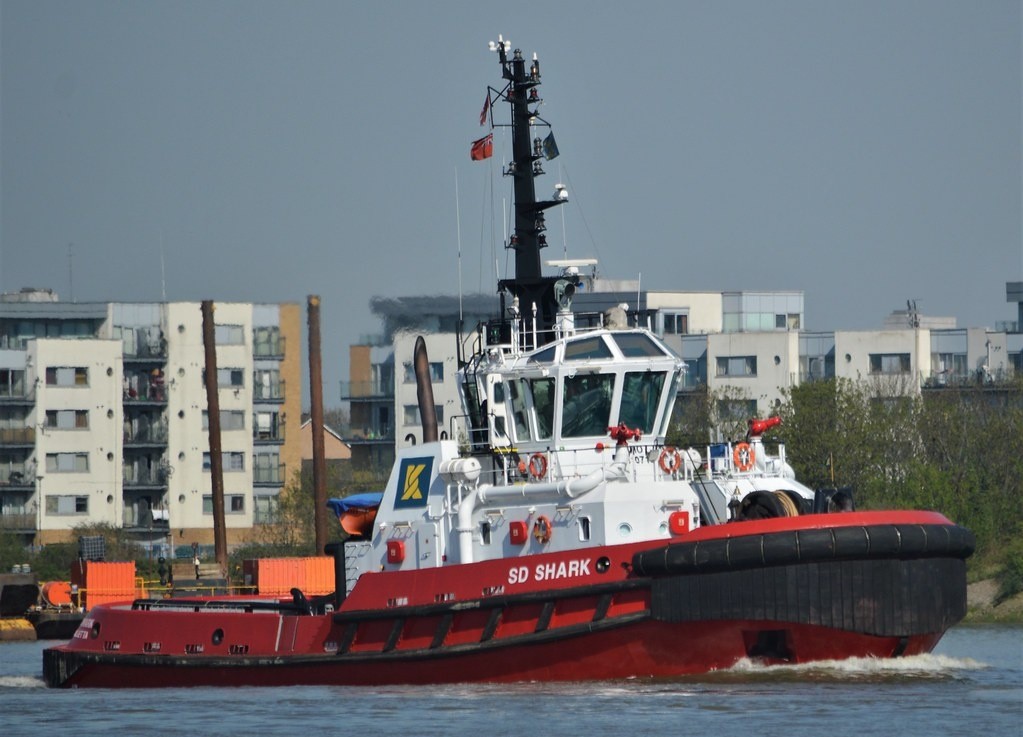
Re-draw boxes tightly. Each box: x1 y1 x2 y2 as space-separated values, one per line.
660 448 680 473
534 515 551 543
734 442 755 471
530 454 546 478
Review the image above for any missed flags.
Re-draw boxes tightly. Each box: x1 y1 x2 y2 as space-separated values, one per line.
480 95 490 126
541 130 559 161
471 133 493 161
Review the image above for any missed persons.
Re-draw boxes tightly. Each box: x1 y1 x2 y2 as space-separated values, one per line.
193 553 200 580
604 303 629 330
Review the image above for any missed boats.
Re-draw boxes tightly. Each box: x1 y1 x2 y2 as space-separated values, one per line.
23 604 88 640
43 34 976 689
